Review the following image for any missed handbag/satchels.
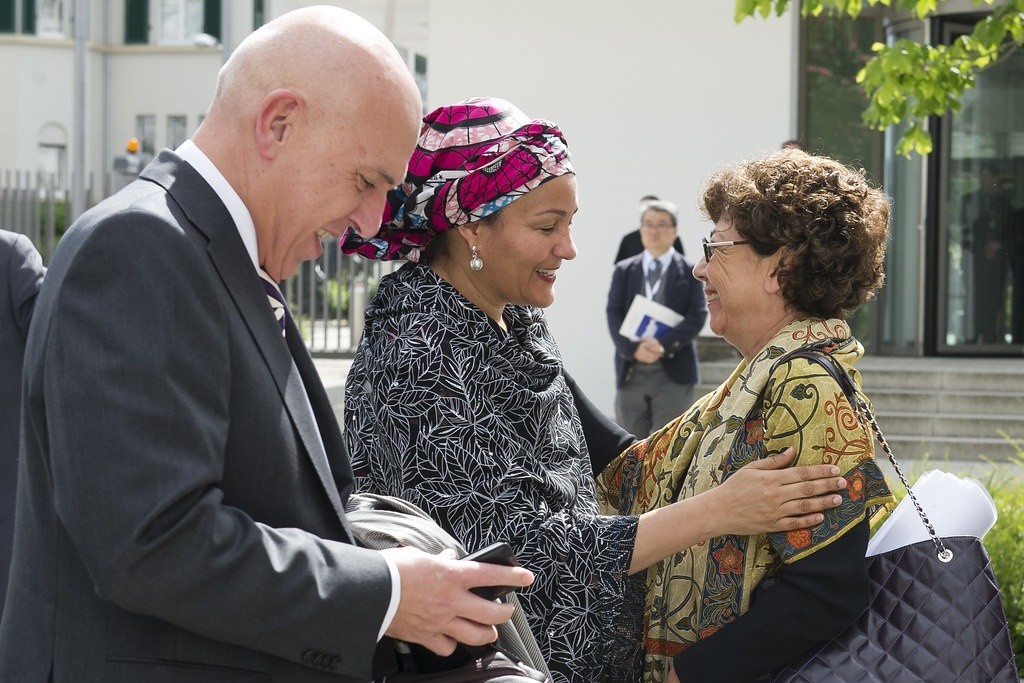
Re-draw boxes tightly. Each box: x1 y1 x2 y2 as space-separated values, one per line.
746 349 1018 683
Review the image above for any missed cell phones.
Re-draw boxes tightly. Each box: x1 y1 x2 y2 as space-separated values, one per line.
458 540 522 601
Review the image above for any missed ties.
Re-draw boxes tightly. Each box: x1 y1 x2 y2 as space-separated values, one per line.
648 259 663 288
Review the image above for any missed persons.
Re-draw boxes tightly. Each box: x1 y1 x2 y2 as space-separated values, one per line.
959 160 1013 349
0 7 537 683
779 141 803 151
592 147 898 683
0 230 49 616
605 196 708 440
343 94 848 683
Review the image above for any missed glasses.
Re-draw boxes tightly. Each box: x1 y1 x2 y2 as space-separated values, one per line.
701 236 789 263
